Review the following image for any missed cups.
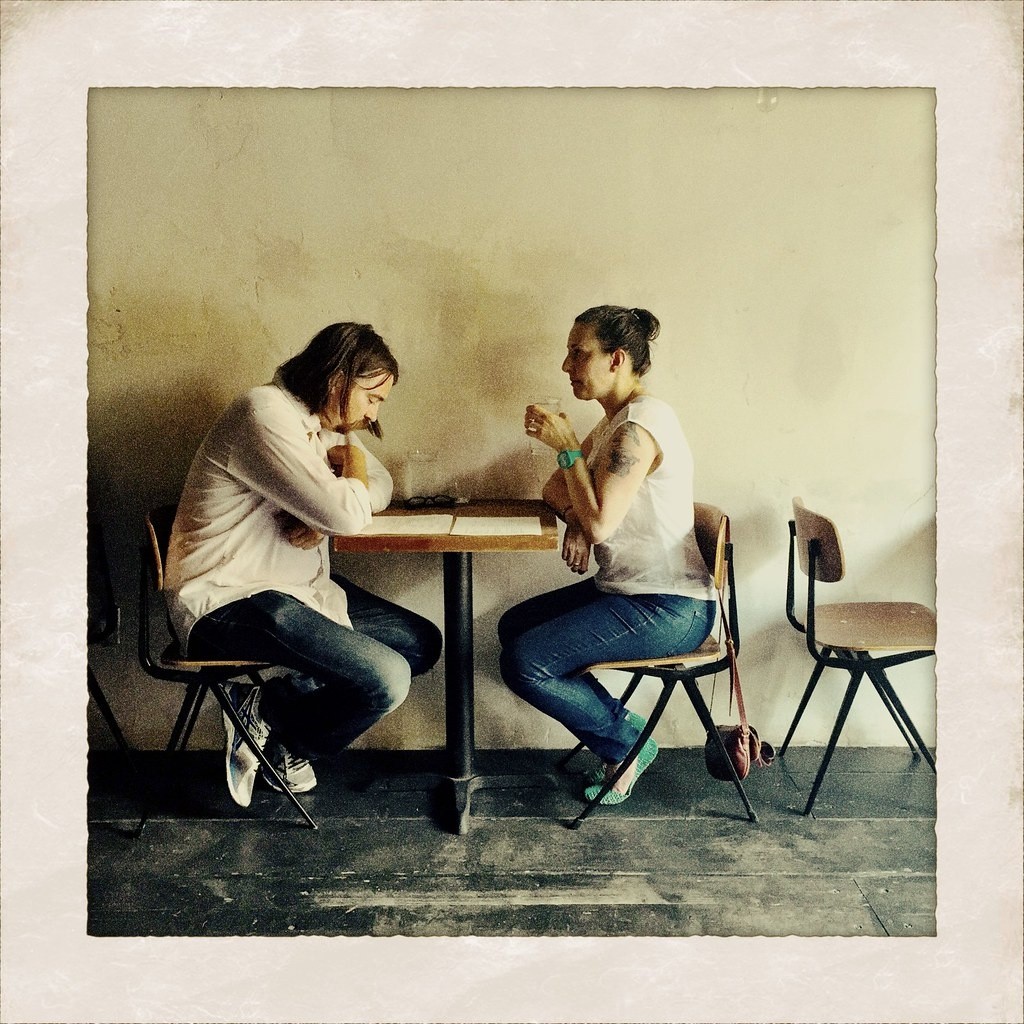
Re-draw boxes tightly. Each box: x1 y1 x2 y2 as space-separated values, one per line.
528 394 560 456
448 482 473 504
407 447 439 498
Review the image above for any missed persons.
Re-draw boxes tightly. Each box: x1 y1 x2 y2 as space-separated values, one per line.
164 321 443 808
497 306 717 806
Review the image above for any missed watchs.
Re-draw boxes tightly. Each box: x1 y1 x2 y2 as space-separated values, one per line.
558 448 585 467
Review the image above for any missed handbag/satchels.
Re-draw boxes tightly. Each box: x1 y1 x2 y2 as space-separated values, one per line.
704 723 776 781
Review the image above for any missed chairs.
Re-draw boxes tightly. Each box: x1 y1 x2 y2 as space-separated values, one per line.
140 503 318 833
778 498 934 820
553 501 758 832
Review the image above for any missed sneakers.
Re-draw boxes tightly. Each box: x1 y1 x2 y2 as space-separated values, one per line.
220 681 318 807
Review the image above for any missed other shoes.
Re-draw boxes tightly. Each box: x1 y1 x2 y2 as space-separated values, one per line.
583 713 659 806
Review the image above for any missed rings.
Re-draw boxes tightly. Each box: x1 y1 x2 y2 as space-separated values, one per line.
573 562 579 566
527 419 535 426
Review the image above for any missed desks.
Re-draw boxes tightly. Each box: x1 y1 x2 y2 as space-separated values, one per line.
332 501 562 824
